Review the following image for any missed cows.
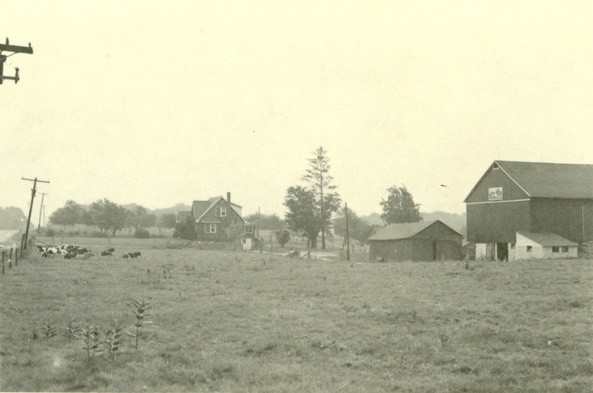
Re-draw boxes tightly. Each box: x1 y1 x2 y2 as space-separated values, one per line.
101 248 115 255
122 251 141 259
36 245 94 260
